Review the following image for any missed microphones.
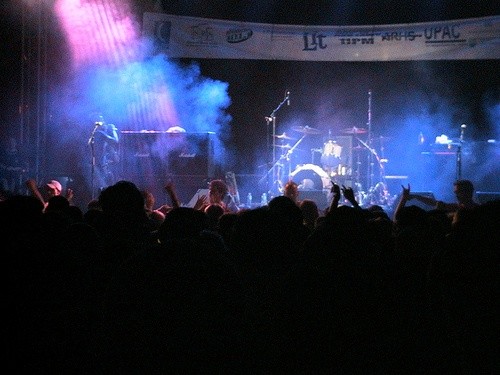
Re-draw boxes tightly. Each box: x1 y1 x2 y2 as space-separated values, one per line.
287 91 290 105
95 122 102 125
265 117 272 121
460 124 466 140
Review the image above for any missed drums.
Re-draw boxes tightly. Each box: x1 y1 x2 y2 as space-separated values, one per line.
330 163 353 181
289 164 331 191
319 138 345 168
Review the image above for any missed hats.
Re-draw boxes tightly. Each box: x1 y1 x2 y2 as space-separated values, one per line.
46 180 62 193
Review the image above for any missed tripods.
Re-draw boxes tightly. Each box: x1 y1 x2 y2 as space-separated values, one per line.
373 138 390 207
261 153 287 197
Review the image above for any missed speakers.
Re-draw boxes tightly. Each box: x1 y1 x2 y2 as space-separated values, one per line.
297 191 329 210
390 192 436 211
120 130 216 210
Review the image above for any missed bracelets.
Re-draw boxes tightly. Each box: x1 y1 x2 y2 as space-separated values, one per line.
330 192 339 200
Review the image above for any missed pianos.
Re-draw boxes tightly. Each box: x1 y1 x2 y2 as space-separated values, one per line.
118 130 216 204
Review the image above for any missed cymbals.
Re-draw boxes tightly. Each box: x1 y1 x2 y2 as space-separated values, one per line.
289 125 323 136
338 127 368 135
271 134 299 142
270 144 304 152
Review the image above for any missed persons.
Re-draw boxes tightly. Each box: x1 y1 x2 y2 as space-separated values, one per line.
0 179 500 375
89 113 119 198
